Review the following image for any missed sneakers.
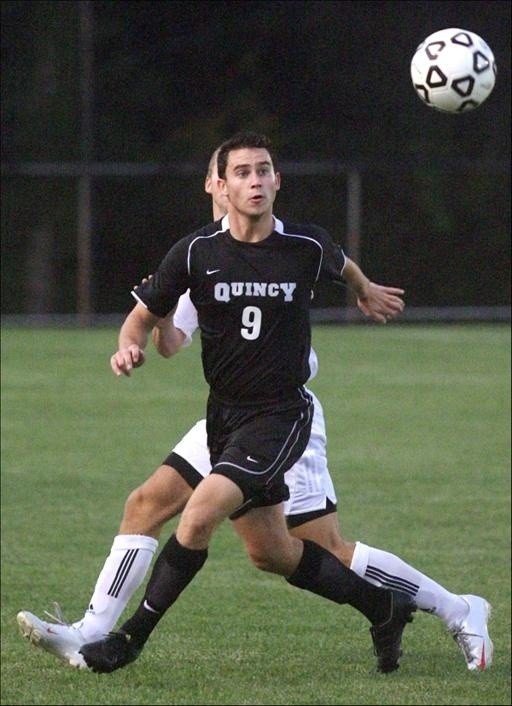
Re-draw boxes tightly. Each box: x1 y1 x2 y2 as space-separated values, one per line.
368 590 417 674
16 611 99 670
445 594 493 674
78 630 144 673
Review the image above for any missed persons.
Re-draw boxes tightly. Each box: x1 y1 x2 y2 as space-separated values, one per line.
76 131 420 676
16 139 496 673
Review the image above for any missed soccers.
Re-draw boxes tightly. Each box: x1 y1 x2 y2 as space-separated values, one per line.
410 27 497 112
